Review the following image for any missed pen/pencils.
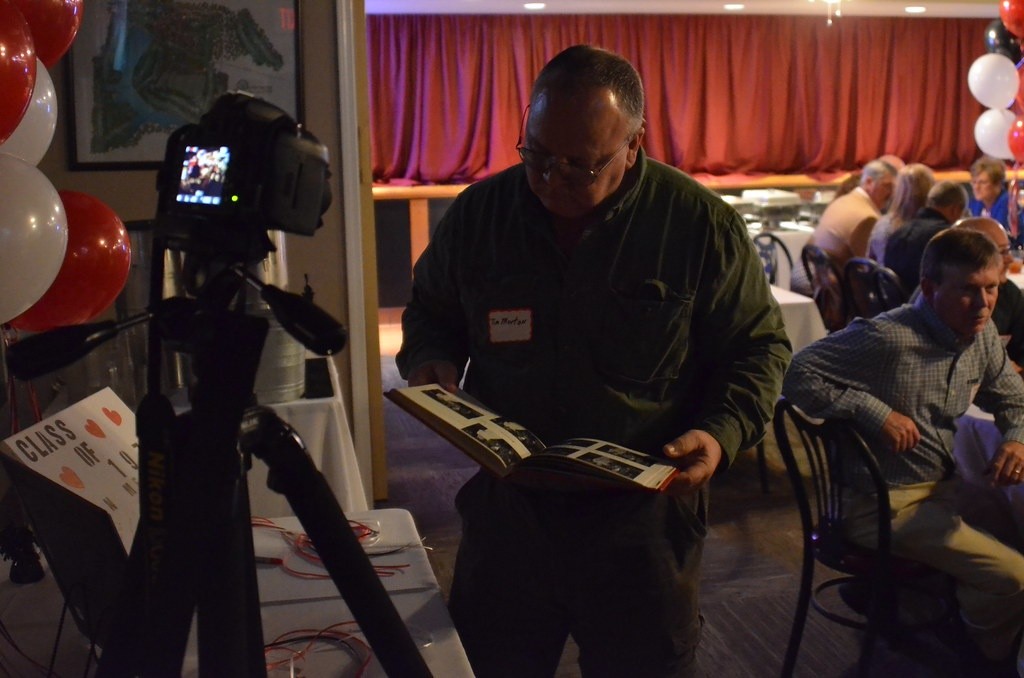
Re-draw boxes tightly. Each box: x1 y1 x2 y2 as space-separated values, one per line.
255 557 282 564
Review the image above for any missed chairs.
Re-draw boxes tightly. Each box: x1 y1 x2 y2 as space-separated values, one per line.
773 396 959 678
753 234 905 328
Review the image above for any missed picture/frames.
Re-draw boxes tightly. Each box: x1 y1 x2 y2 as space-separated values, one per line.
61 2 305 168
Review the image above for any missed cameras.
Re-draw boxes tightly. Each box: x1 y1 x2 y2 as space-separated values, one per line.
156 91 333 236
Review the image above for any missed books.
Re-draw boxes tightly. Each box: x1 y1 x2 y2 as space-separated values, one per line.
382 385 682 495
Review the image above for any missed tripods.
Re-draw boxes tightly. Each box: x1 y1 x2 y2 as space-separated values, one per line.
3 216 436 678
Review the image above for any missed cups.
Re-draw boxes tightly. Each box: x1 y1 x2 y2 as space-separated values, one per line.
1006 248 1024 273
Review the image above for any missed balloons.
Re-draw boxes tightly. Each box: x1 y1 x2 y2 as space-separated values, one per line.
967 0 1024 166
0 0 131 333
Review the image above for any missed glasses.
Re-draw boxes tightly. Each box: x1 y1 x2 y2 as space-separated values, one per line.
1002 246 1016 255
516 105 633 188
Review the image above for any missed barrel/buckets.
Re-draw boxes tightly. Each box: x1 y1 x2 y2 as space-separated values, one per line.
248 306 308 405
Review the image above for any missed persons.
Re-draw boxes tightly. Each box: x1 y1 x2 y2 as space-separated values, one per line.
779 226 1024 678
394 43 793 678
812 151 1024 528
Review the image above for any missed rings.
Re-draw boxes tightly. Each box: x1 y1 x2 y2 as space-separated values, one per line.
1013 469 1021 474
